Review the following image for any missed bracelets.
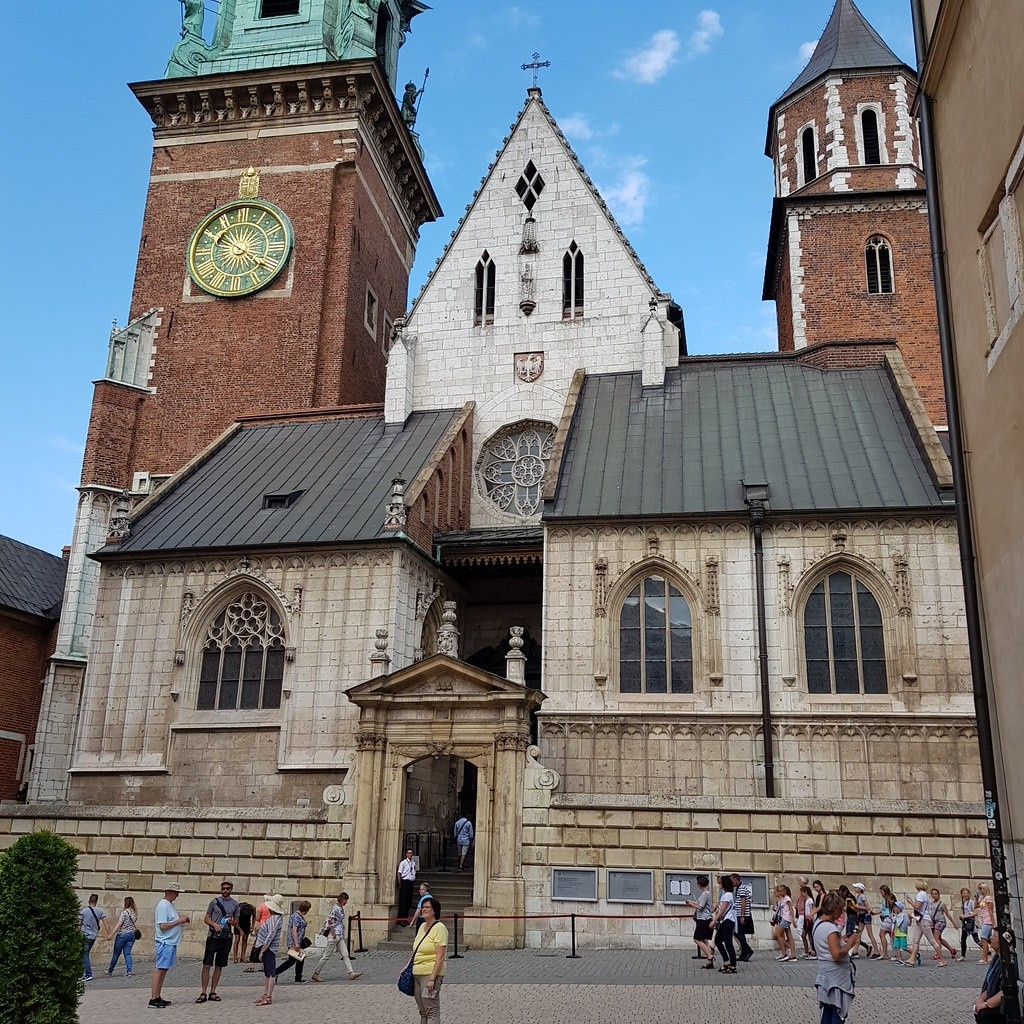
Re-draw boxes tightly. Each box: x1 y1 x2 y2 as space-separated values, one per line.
985 1001 990 1008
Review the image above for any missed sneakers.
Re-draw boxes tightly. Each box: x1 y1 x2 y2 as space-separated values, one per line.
148 996 171 1009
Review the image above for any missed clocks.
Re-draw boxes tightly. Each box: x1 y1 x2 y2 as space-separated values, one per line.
189 198 291 300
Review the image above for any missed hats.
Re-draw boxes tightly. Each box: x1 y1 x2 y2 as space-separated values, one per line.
851 882 866 893
220 880 234 886
894 900 905 911
264 892 286 916
161 881 187 892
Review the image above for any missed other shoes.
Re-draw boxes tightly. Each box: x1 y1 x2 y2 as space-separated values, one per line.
456 866 464 872
699 949 754 973
851 945 995 968
103 969 131 977
77 975 93 983
774 953 818 963
232 957 265 973
294 971 363 985
398 921 412 927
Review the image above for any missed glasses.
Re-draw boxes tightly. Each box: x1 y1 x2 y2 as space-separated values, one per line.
221 886 233 890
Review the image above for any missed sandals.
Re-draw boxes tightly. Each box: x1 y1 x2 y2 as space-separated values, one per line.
195 993 221 1003
253 994 272 1007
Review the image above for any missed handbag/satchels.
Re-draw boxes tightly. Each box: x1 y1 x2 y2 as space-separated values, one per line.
963 919 974 930
317 919 331 937
692 909 698 921
714 920 721 930
134 928 142 941
802 919 815 930
909 910 922 922
769 911 781 926
396 957 415 996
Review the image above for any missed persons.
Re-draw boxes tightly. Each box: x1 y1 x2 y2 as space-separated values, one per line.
400 897 450 1024
769 884 799 962
195 880 240 1003
275 901 311 984
403 83 423 120
312 892 362 982
957 883 996 965
243 889 285 1006
397 848 417 927
971 926 1018 1024
103 897 138 977
77 894 111 982
453 814 474 872
686 874 754 974
812 894 859 1024
147 882 190 1008
796 875 959 967
233 902 256 963
409 882 434 938
